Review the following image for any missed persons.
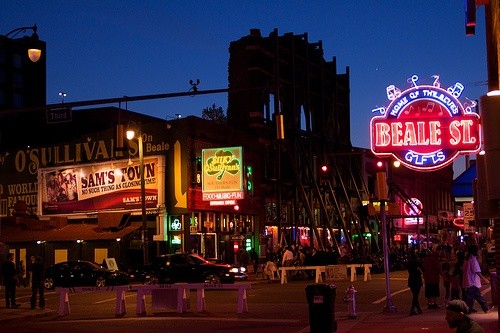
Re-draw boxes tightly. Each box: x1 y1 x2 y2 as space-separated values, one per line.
405 231 495 316
17 260 27 288
443 298 488 333
2 252 22 310
28 255 48 312
217 240 407 278
47 171 76 203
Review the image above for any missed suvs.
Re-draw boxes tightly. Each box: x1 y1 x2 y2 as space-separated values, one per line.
150 253 229 285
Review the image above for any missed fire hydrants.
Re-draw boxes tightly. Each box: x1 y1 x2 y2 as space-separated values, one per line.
342 283 360 322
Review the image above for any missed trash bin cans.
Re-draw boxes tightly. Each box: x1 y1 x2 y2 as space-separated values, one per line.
305 283 338 333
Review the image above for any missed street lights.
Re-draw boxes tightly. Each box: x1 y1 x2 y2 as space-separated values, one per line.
366 197 400 313
124 120 149 270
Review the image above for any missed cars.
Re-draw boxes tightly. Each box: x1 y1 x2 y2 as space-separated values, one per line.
41 260 132 290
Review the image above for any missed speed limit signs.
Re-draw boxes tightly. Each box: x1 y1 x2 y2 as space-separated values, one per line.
464 203 476 221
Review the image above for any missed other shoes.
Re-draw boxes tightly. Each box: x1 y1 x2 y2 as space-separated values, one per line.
469 308 478 313
427 303 434 309
484 306 494 313
12 304 21 308
6 304 10 308
432 304 440 309
40 306 45 310
30 306 36 310
416 309 423 314
410 310 417 315
